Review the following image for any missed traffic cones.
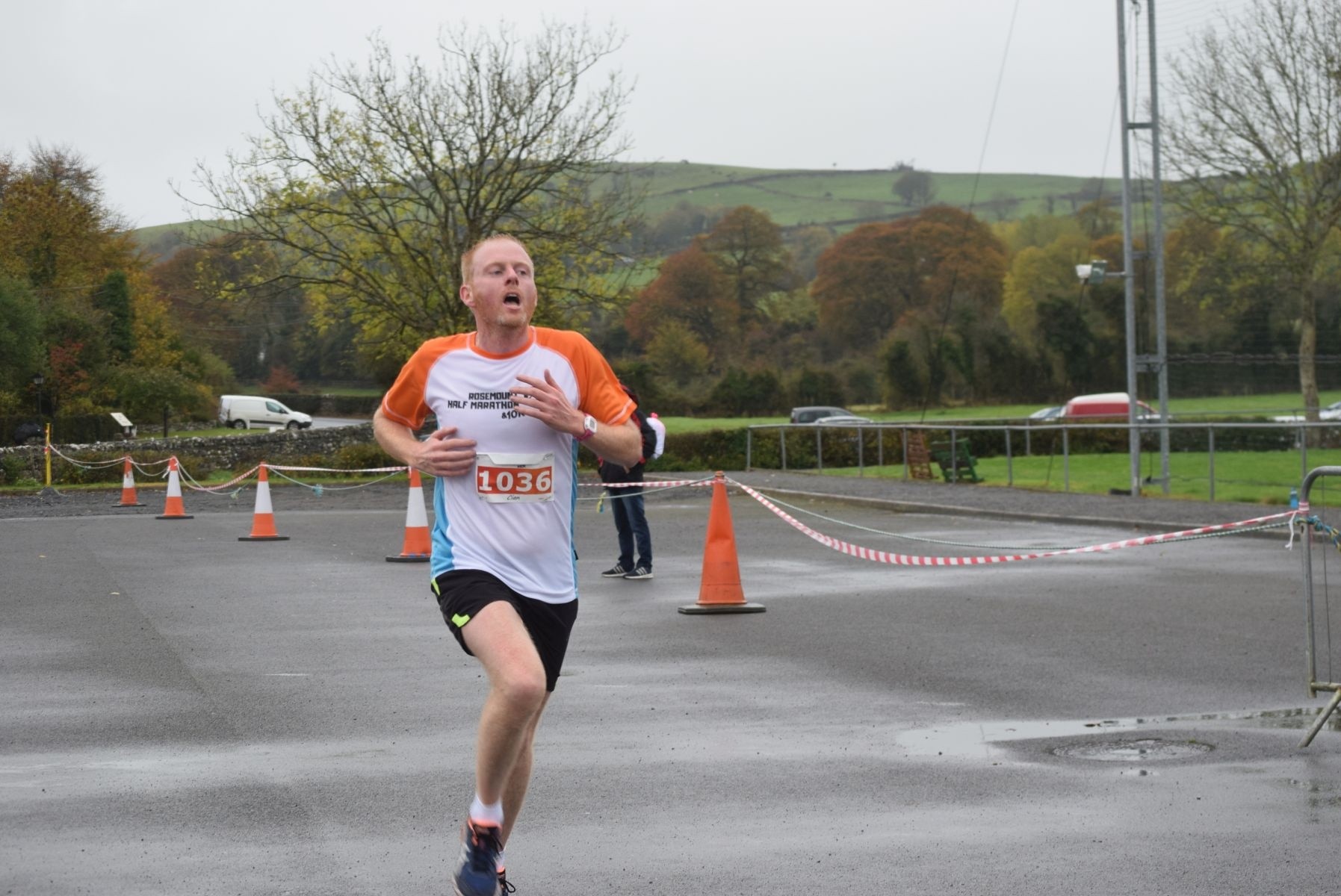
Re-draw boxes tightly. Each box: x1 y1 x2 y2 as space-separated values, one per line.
678 473 767 613
386 468 432 563
113 457 147 508
238 465 289 541
153 458 194 519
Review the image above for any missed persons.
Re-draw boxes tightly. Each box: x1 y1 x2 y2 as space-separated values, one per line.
371 230 640 896
599 375 654 581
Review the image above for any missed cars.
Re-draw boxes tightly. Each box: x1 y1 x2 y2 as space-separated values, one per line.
791 407 877 426
1029 392 1171 424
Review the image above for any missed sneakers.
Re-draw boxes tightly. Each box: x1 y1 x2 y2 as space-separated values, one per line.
601 561 635 578
622 564 654 579
452 811 505 896
491 866 516 896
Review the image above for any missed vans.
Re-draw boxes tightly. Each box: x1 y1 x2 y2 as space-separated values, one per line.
217 395 313 430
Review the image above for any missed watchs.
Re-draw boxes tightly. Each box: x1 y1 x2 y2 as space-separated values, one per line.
574 412 599 441
